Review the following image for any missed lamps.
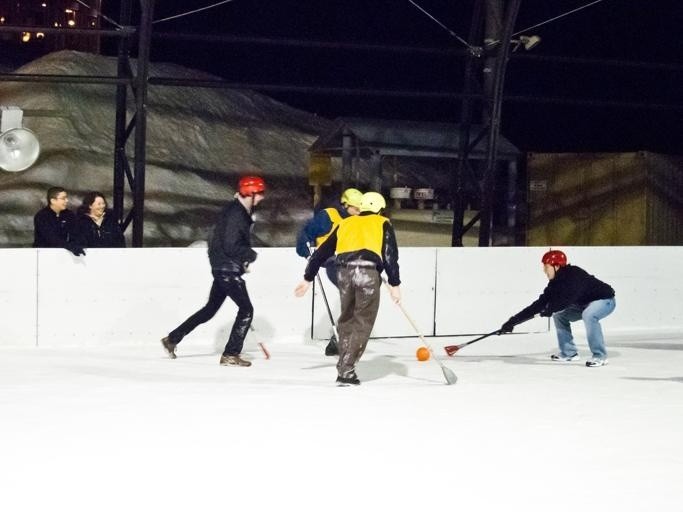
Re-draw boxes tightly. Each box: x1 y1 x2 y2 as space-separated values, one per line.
0 100 41 174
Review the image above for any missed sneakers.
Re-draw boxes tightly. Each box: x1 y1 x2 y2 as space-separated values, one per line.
551 352 581 361
586 356 607 365
220 354 254 366
337 373 359 386
324 344 338 353
160 336 179 360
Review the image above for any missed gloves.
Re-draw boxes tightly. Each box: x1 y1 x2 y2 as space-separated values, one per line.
540 305 553 315
501 321 512 332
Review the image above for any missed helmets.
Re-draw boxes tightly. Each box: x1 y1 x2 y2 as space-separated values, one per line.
542 250 569 267
341 187 363 209
359 191 387 213
238 175 266 197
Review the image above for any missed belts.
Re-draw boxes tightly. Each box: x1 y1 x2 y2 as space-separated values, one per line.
338 262 379 272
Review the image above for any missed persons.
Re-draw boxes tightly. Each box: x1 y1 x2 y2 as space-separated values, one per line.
295 187 367 355
59 191 126 258
31 186 75 248
495 249 617 368
159 173 266 369
288 191 403 388
